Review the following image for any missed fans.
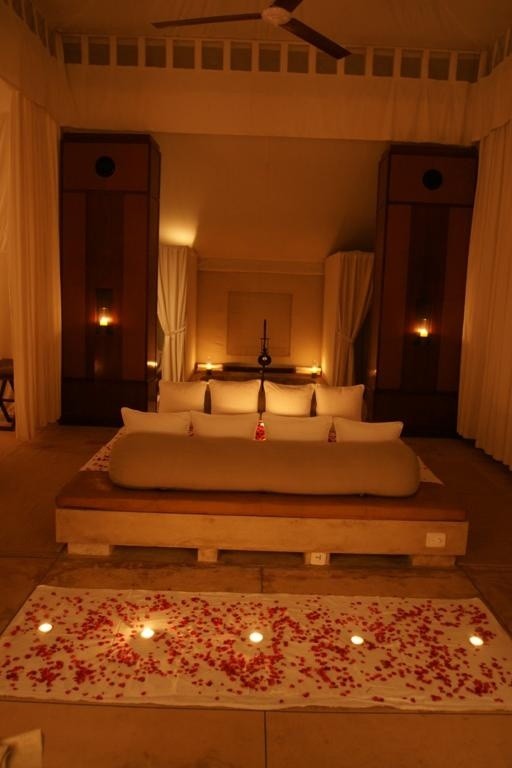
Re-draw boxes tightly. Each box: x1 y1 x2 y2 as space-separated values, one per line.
149 0 352 60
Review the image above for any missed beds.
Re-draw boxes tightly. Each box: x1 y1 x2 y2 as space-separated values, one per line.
55 392 468 569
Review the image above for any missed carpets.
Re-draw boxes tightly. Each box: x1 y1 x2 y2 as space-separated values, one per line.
0 585 512 711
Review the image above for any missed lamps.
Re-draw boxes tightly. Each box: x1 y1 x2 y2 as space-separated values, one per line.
94 288 113 334
414 299 434 353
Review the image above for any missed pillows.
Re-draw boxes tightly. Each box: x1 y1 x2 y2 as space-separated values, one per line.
191 409 260 441
208 381 260 413
262 380 315 415
261 411 332 441
120 406 192 436
316 383 365 421
157 380 206 413
333 417 404 443
